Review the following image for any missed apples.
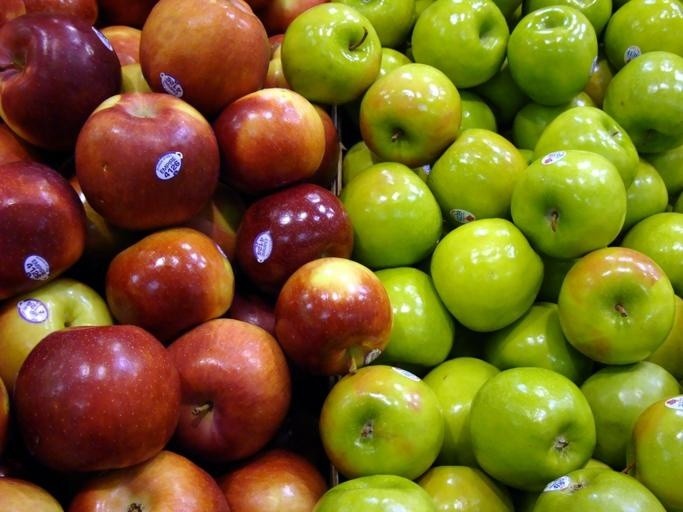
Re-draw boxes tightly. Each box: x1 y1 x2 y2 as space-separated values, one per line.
0 0 683 512
